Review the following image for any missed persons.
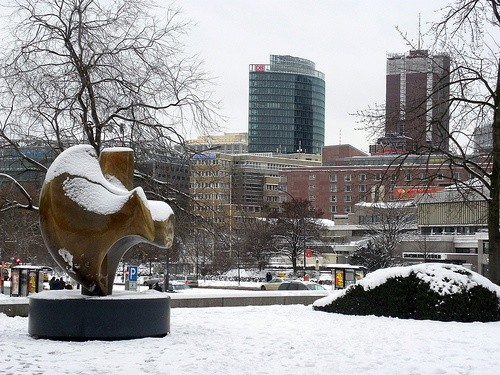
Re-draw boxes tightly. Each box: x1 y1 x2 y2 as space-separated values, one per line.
266 271 310 282
154 282 163 292
3 269 73 290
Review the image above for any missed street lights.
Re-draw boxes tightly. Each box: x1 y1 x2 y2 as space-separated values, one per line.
277 187 306 276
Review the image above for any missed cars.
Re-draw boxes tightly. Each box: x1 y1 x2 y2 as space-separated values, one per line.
277 280 327 290
260 277 285 291
185 275 199 288
